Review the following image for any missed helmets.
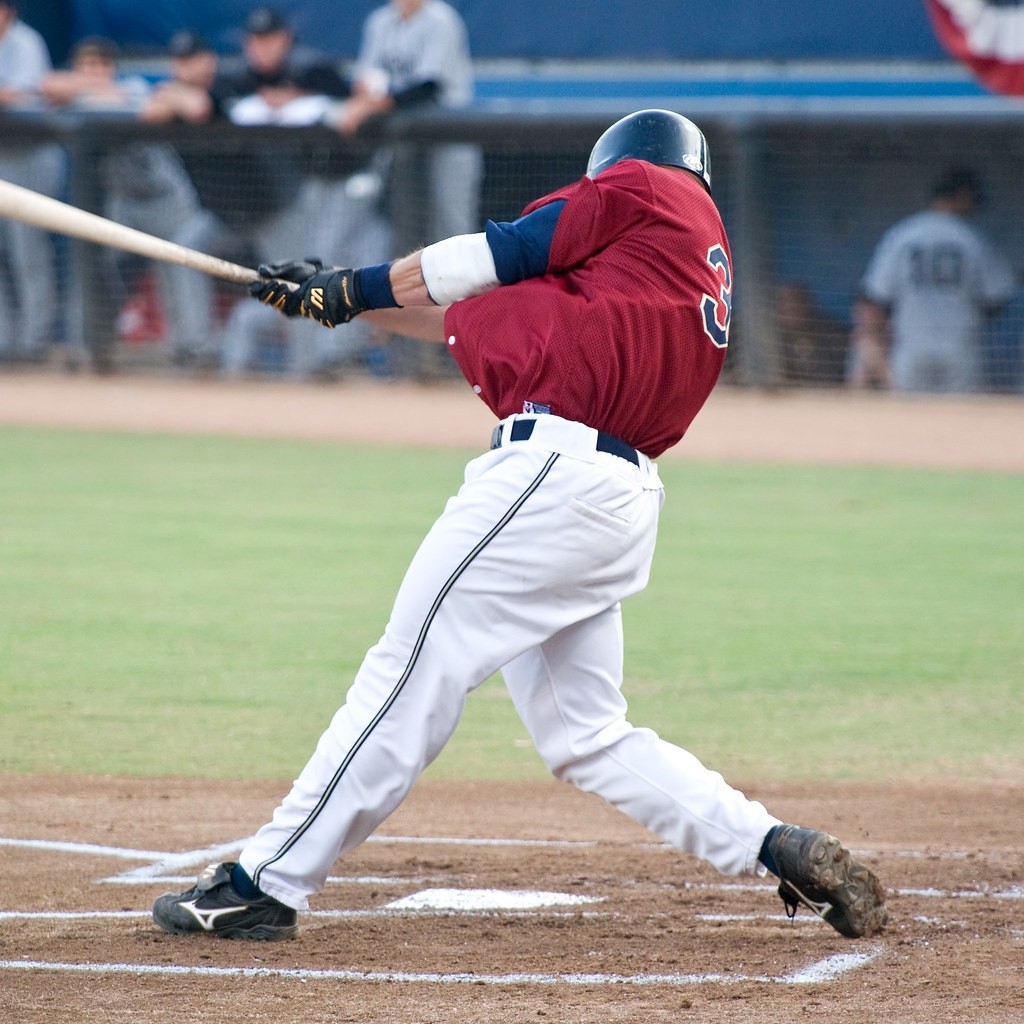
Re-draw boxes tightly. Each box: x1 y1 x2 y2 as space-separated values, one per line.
588 108 713 197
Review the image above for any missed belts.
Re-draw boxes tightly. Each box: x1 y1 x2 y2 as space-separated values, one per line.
489 420 639 468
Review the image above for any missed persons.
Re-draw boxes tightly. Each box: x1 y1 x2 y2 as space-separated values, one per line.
338 0 485 245
1 0 393 374
152 110 888 938
852 166 1019 393
777 283 848 387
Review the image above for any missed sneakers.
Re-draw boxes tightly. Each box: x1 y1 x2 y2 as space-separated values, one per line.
768 823 889 938
152 862 301 941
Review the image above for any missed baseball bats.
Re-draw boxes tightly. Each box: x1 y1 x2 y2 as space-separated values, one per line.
0 179 303 295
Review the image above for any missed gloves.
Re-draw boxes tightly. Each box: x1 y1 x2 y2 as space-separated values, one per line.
246 258 369 331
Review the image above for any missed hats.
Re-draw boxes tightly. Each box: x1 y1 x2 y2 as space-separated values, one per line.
226 9 286 37
923 154 992 208
161 31 210 57
